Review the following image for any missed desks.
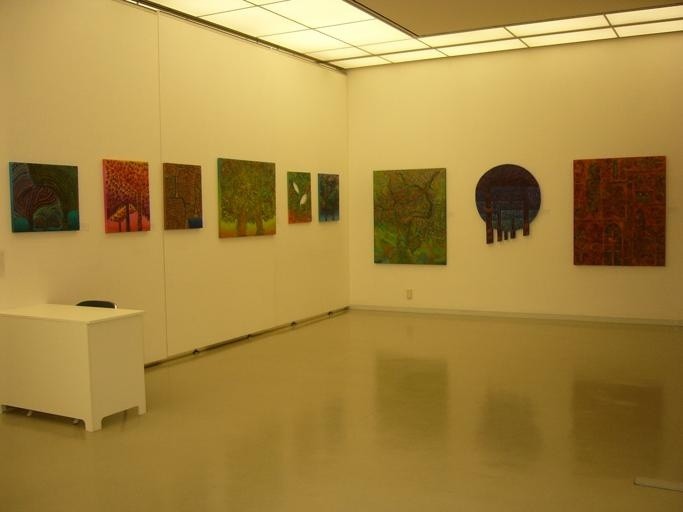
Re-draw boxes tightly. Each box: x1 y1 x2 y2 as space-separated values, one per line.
0 305 146 433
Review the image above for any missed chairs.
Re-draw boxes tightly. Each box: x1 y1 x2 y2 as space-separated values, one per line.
76 300 118 308
